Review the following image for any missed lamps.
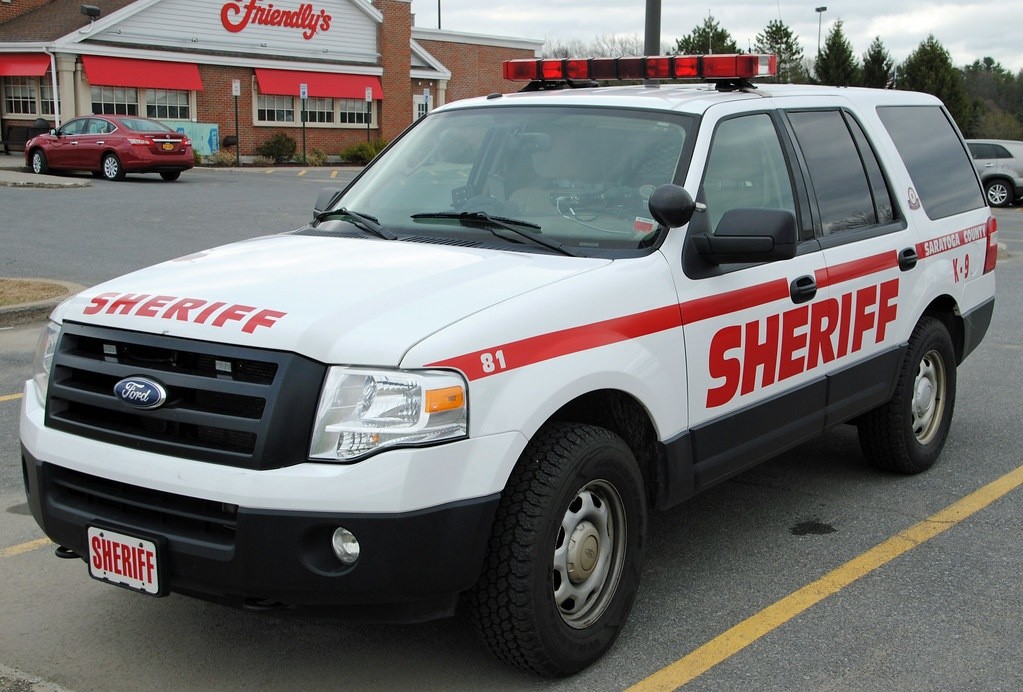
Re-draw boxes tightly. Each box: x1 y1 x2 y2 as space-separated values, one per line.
79 4 101 20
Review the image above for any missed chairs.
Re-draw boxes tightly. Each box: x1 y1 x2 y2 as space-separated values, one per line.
508 122 779 235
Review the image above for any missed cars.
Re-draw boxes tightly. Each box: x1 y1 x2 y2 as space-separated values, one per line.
25 114 196 182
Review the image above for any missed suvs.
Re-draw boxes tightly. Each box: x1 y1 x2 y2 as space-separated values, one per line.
20 53 998 680
965 138 1023 208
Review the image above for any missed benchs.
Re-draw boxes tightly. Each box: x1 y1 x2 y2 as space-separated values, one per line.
0 125 56 154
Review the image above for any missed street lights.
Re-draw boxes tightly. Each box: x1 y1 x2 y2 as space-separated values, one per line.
815 5 828 56
